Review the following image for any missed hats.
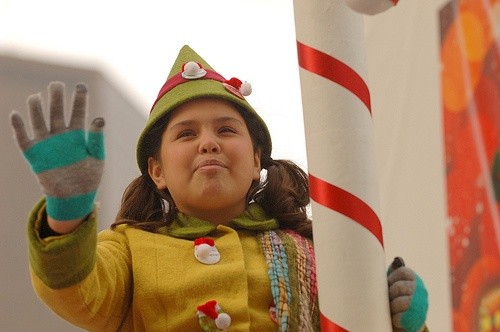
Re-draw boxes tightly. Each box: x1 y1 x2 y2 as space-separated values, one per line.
136 44 272 173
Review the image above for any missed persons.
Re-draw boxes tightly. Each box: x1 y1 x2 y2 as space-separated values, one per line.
9 45 430 332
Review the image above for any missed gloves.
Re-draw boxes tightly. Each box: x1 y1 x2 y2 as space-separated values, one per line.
387 254 429 332
10 80 106 222
